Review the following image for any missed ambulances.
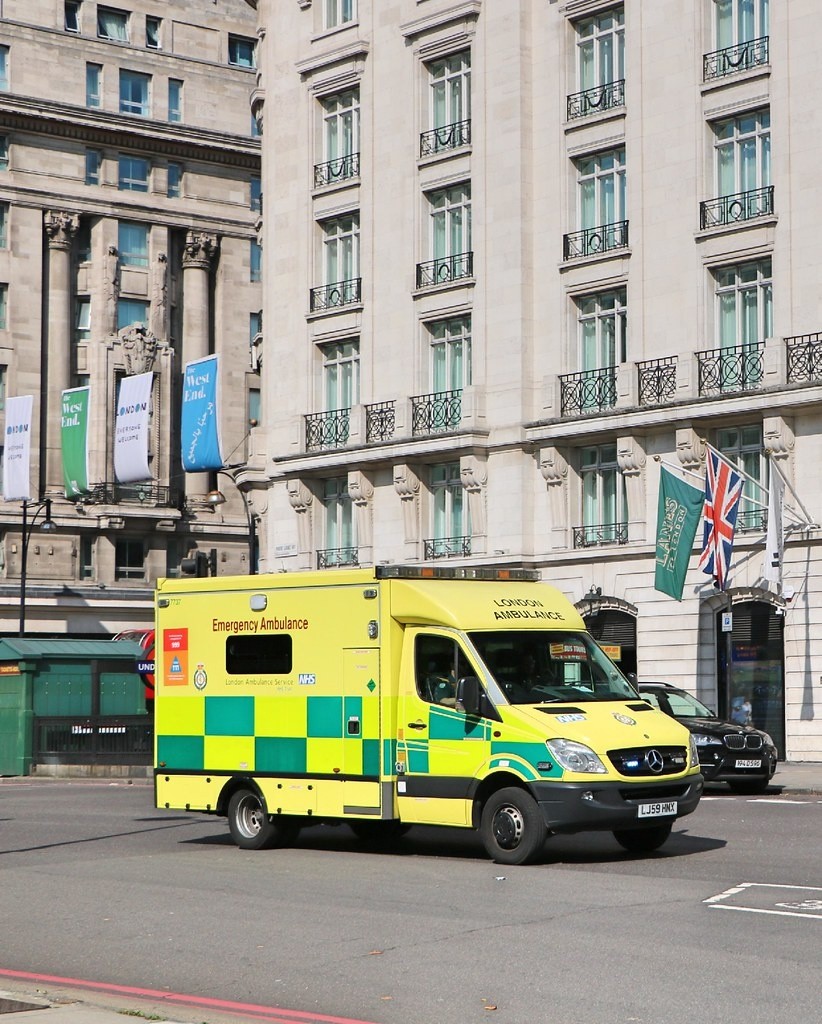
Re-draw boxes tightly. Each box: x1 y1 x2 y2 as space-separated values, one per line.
147 555 703 868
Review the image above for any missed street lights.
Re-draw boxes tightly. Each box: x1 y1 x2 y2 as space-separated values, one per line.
18 498 57 637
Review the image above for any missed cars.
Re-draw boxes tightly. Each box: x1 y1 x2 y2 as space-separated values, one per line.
634 679 780 796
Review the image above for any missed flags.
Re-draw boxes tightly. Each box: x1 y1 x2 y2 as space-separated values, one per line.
655 465 705 604
699 443 746 594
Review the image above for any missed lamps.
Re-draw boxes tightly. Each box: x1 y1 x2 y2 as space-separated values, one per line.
582 584 603 612
73 503 85 510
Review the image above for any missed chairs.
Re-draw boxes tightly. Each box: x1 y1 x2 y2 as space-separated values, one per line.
424 662 450 704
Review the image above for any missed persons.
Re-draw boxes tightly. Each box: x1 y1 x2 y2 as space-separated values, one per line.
512 645 538 684
433 652 472 707
